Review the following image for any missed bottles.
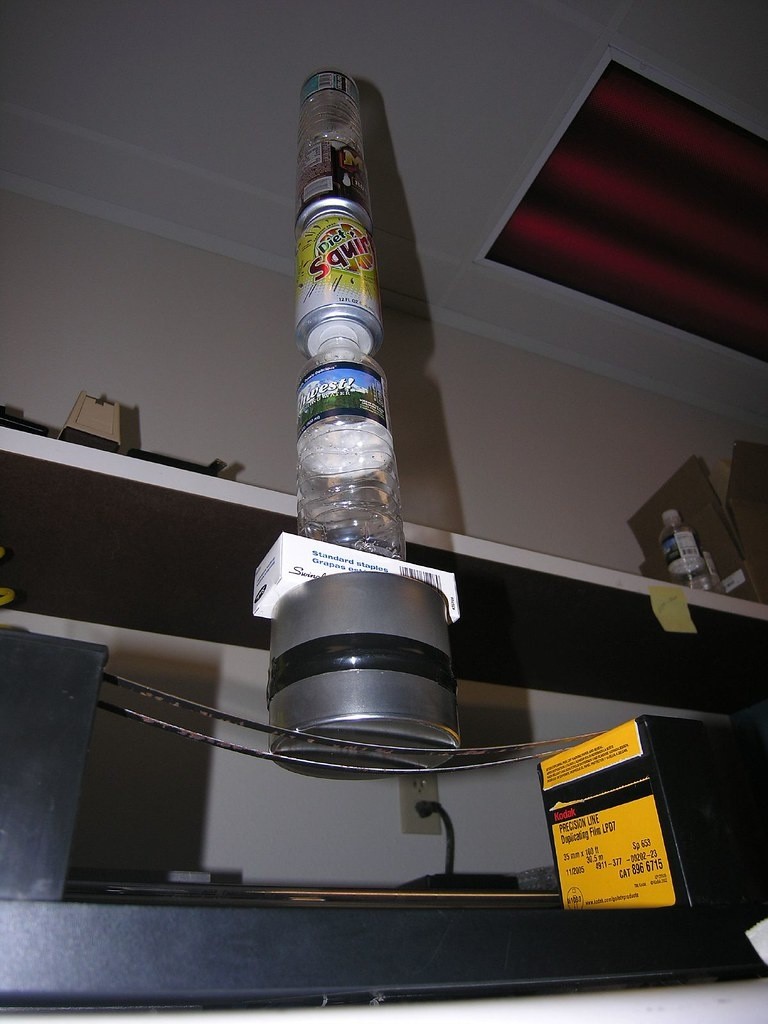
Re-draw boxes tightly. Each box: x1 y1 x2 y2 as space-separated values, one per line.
296 336 407 562
656 509 711 592
298 67 365 158
